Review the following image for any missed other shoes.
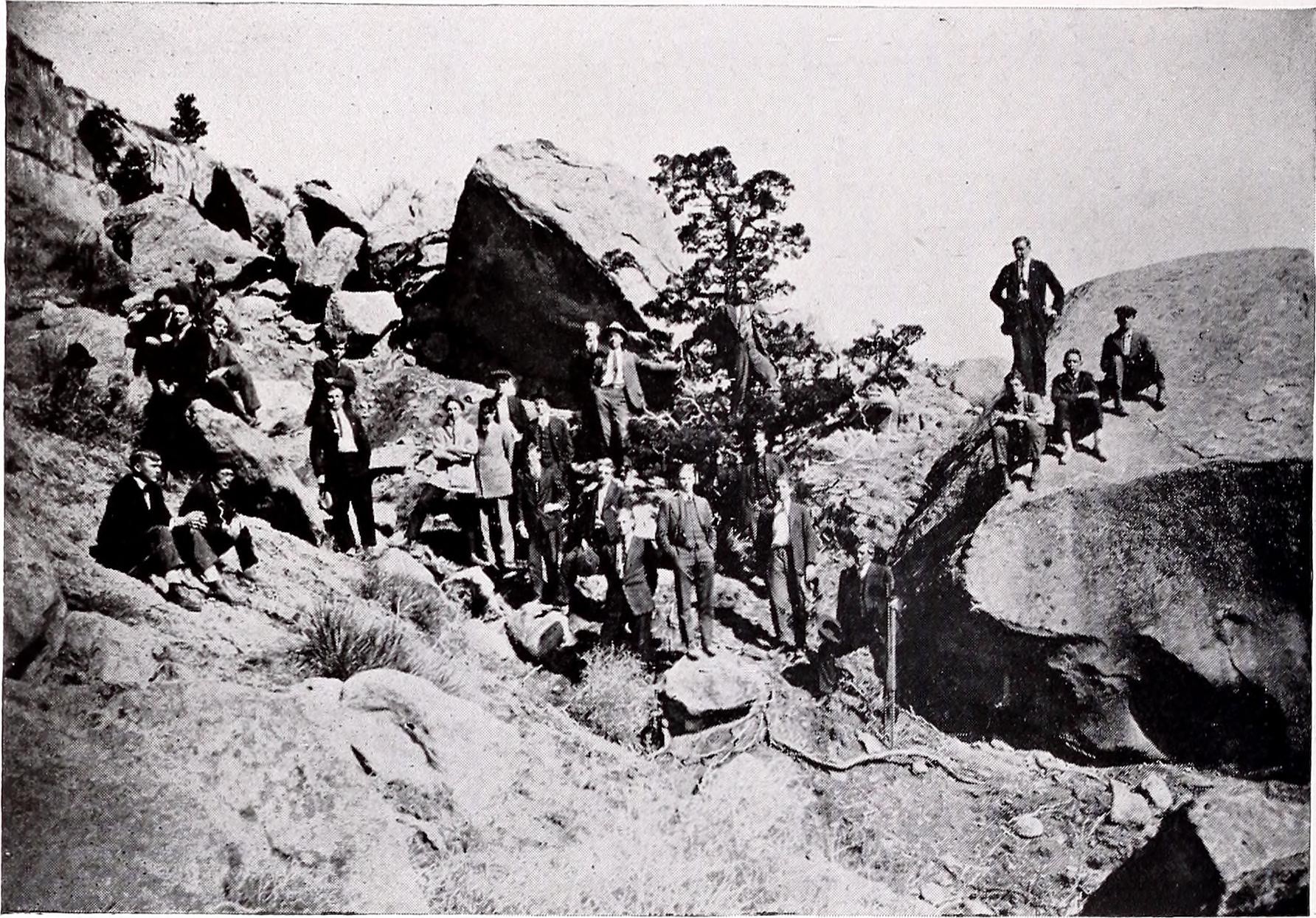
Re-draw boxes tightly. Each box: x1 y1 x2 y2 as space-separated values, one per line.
706 640 716 655
243 413 262 426
1061 449 1075 465
347 545 360 558
364 545 379 560
1155 394 1167 410
1114 402 1128 416
168 583 201 611
769 642 785 658
1000 477 1012 496
1093 447 1108 463
687 644 699 661
399 537 411 547
1030 468 1043 491
209 580 237 603
818 693 830 711
469 553 489 567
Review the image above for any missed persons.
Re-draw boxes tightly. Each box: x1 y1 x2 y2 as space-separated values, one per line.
810 536 901 726
95 260 263 612
304 334 382 558
988 235 1167 494
384 320 719 662
737 429 818 658
710 278 780 416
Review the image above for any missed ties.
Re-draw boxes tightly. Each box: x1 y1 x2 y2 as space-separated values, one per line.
613 351 618 384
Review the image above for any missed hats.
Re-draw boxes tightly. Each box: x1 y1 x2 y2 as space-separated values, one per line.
1114 305 1136 317
210 451 241 475
818 621 844 645
488 365 512 382
604 321 630 340
